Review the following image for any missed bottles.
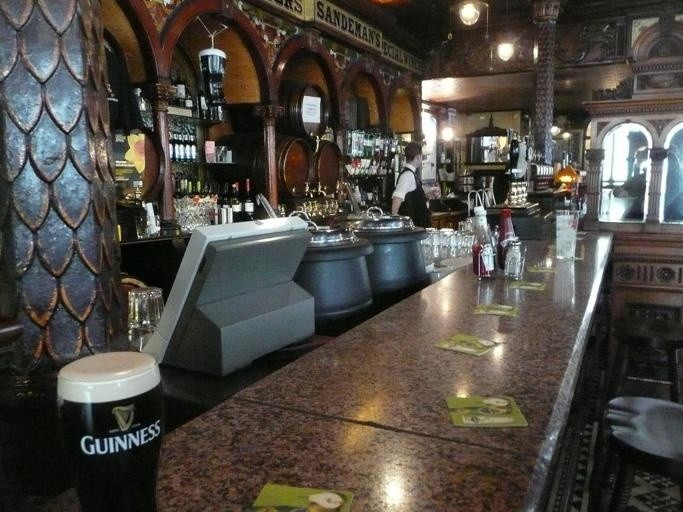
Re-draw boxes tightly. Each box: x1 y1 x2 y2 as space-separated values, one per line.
172 178 218 198
166 131 198 163
217 178 254 224
347 127 398 176
472 206 527 281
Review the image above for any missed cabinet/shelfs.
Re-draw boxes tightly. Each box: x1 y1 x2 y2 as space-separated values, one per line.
167 103 237 199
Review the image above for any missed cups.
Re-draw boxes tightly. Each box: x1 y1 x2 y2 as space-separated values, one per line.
555 210 580 259
421 222 472 265
129 288 163 329
54 351 163 508
133 214 152 241
553 260 576 309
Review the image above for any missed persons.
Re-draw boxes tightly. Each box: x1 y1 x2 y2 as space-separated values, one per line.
611 174 648 200
388 141 433 230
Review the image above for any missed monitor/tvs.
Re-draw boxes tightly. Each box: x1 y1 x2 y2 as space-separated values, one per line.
140 216 316 378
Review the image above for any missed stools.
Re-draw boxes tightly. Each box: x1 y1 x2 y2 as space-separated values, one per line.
602 316 683 403
595 396 683 512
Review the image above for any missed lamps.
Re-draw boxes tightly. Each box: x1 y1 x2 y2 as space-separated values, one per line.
497 0 514 62
449 0 489 26
